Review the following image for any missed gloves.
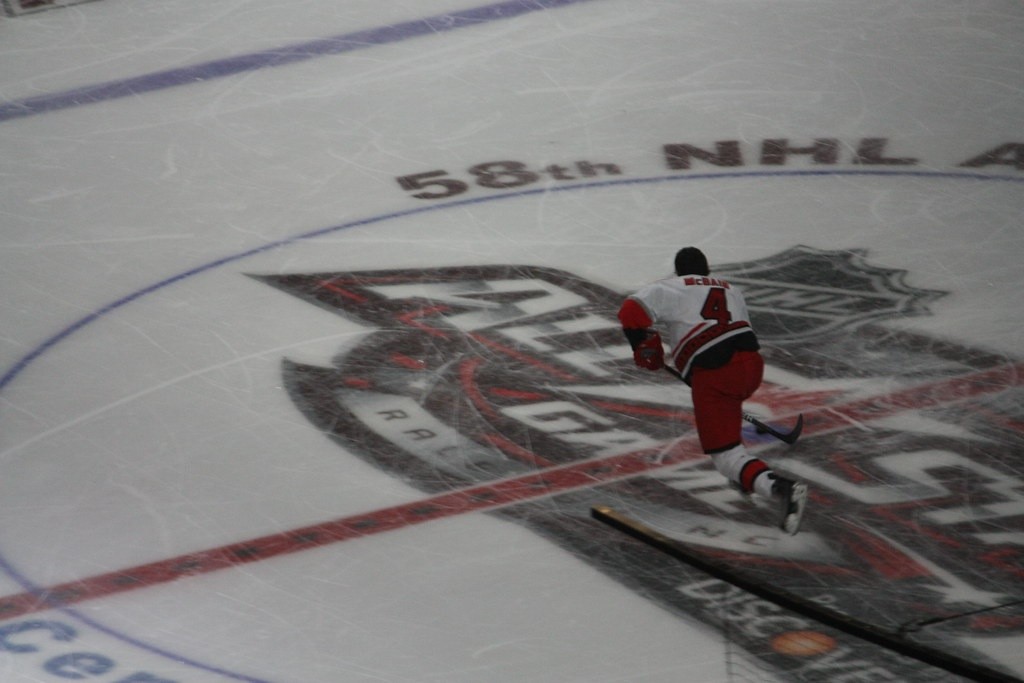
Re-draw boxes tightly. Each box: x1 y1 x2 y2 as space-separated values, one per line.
633 330 665 372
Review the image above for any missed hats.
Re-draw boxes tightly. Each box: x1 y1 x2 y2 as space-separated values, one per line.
675 246 709 273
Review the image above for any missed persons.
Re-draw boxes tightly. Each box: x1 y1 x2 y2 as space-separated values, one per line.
619 244 810 539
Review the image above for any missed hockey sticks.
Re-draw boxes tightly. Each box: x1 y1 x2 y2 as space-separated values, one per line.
662 362 804 445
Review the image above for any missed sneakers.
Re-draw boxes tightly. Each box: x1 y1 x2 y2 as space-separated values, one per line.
769 472 804 535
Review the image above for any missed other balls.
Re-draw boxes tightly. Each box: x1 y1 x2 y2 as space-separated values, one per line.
757 426 767 434
